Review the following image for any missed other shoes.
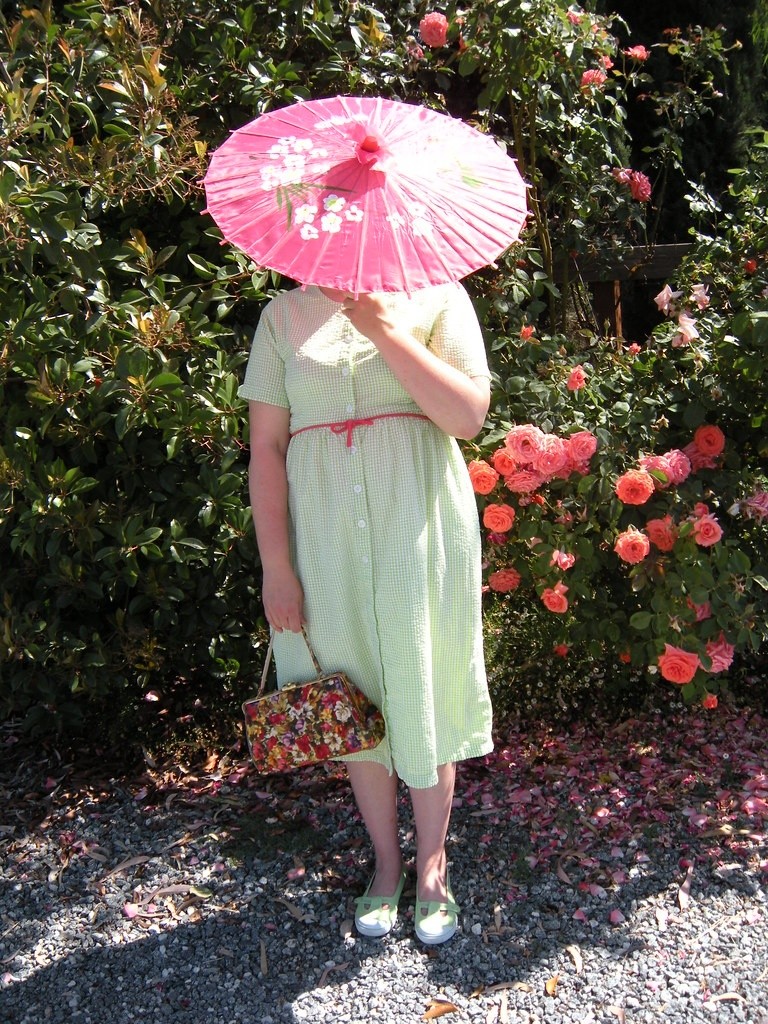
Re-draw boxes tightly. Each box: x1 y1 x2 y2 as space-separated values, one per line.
413 867 461 946
353 865 405 937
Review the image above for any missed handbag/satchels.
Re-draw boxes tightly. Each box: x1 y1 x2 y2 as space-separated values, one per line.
240 622 386 775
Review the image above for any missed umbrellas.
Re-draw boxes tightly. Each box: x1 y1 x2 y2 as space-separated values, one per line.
197 94 537 301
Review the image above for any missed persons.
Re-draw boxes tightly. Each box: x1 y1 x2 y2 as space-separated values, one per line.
238 283 494 946
